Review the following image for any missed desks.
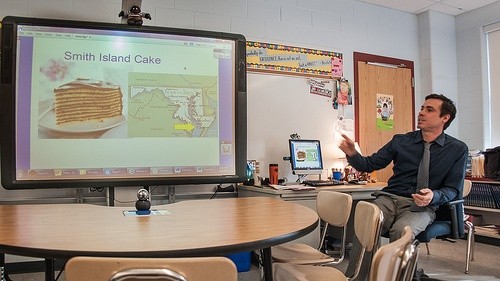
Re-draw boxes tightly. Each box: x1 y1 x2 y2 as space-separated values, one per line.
1 197 318 281
236 180 388 255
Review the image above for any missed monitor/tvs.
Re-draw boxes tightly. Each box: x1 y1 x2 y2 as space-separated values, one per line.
1 16 247 191
289 139 324 183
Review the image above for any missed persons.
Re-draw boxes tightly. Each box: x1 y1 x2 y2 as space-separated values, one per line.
339 93 469 281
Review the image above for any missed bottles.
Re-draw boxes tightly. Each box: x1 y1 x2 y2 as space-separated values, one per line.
254 162 261 186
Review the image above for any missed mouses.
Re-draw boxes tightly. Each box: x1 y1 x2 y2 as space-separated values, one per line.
341 180 348 184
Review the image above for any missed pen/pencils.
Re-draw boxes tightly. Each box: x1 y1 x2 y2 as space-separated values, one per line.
332 168 342 172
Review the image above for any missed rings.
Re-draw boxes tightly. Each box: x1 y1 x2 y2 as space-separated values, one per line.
419 202 423 205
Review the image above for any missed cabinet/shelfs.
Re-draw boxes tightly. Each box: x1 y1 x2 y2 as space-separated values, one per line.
462 177 500 247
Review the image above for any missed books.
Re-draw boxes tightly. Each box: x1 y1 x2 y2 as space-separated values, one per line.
463 184 500 208
464 214 500 235
466 151 485 177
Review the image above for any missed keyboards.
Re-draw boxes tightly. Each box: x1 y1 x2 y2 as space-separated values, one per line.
304 179 345 187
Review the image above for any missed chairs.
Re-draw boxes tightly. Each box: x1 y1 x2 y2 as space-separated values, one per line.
426 180 475 273
64 256 238 281
371 186 468 244
271 190 353 267
370 225 420 281
271 201 384 281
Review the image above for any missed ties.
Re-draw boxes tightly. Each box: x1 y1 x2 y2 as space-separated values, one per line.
411 142 433 212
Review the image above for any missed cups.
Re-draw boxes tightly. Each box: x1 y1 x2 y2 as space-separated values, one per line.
334 172 341 180
269 164 278 184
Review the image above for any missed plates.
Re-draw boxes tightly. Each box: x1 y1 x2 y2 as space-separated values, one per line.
38 106 126 133
